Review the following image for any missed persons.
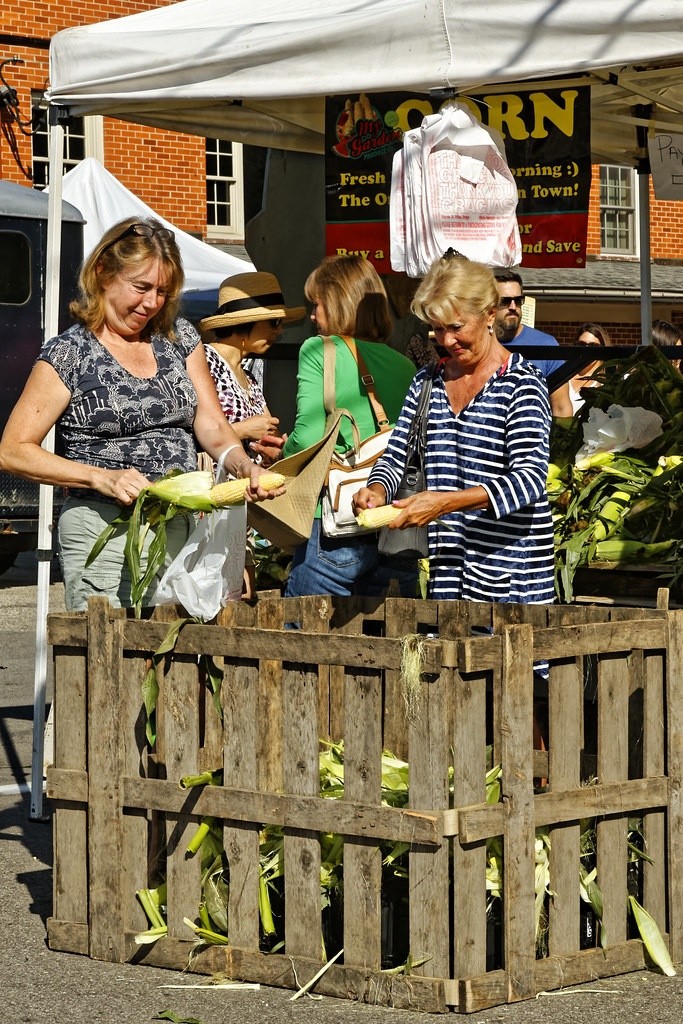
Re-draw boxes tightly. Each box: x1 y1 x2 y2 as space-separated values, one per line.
0 217 682 748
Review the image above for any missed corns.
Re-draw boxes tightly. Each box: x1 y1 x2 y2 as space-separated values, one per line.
210 473 285 504
544 350 683 565
355 503 406 529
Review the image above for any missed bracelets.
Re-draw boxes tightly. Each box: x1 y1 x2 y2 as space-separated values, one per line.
234 458 251 478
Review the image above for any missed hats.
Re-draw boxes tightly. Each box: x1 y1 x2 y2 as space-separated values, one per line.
199 272 306 330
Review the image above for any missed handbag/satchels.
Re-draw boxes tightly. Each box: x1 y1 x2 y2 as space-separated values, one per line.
247 335 362 552
321 428 396 539
148 445 248 622
377 360 439 558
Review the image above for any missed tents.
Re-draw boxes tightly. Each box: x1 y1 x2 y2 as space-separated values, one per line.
33 155 256 291
30 0 682 819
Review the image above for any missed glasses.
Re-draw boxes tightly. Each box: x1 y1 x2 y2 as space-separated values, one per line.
574 340 600 347
498 295 526 308
100 224 175 256
265 318 282 327
